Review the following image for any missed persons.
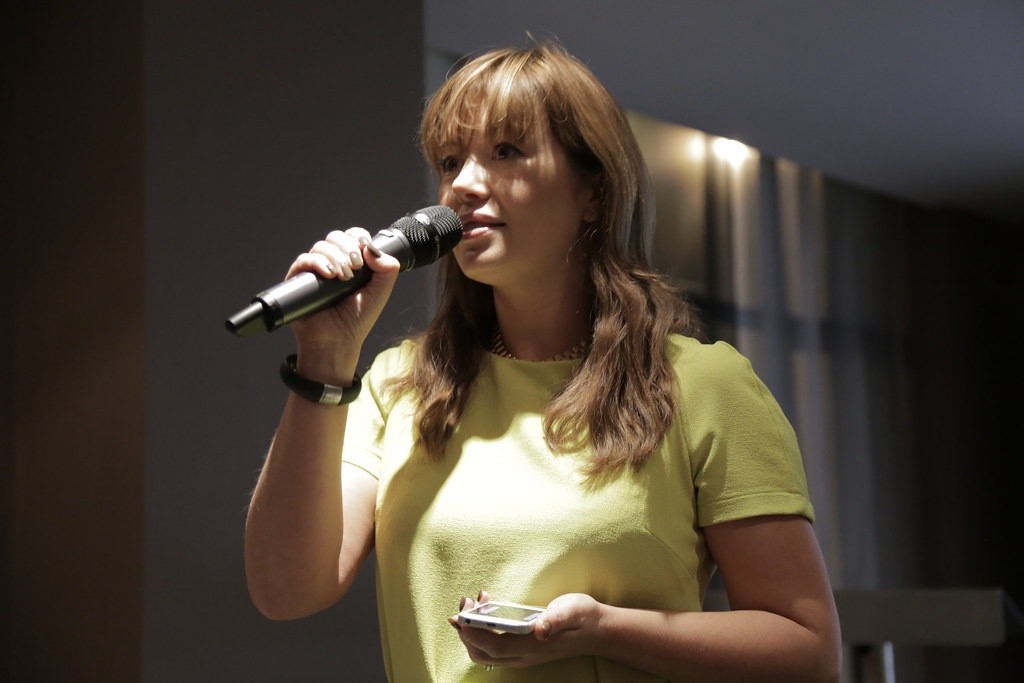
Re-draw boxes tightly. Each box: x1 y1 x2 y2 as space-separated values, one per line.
245 47 841 683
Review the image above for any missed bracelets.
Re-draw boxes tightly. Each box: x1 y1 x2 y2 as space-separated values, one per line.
279 353 362 406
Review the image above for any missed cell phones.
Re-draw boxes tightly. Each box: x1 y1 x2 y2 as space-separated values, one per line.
458 601 546 634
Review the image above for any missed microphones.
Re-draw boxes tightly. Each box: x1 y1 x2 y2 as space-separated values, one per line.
224 205 464 339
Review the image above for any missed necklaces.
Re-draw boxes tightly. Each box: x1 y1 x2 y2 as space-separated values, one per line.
491 329 589 363
